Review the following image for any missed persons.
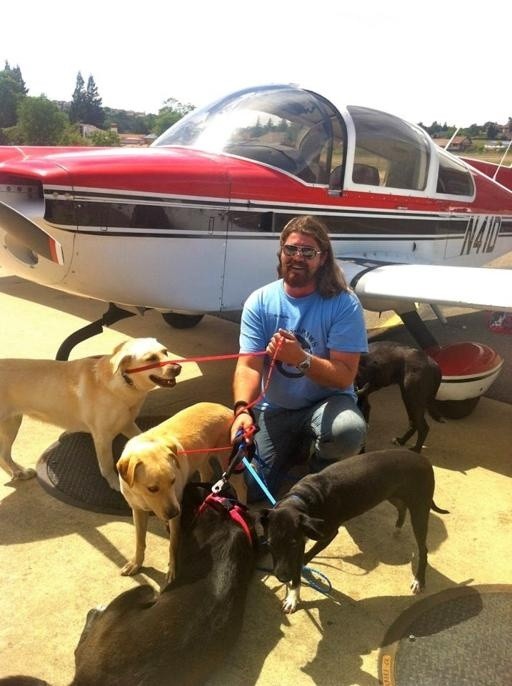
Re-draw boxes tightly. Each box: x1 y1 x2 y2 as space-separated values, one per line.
230 215 370 505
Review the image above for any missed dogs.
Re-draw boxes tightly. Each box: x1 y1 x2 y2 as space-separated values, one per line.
116 402 248 583
0 470 258 686
0 338 182 492
259 449 450 614
354 341 447 454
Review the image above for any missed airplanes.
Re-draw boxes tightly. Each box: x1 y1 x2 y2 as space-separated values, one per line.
0 84 511 419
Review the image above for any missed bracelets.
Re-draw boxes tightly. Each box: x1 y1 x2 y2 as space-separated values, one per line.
238 411 249 416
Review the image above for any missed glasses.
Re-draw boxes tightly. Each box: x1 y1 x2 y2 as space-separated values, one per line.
281 243 322 260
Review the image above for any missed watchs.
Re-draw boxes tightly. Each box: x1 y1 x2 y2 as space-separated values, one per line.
298 354 312 372
